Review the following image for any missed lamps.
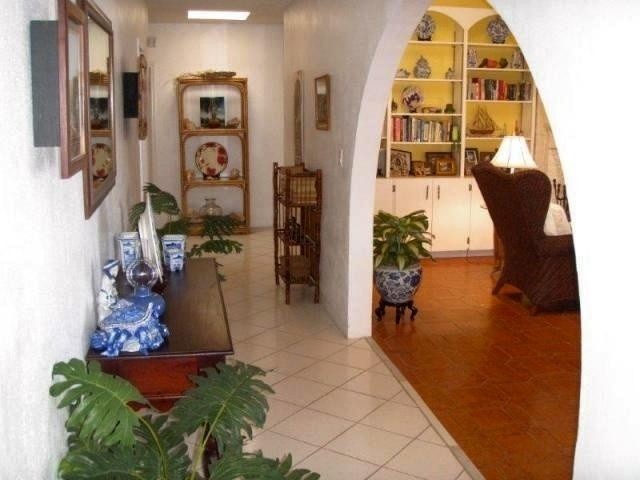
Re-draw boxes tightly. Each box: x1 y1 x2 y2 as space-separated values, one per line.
490 136 539 174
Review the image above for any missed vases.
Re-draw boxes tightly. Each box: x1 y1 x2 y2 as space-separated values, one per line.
117 231 187 273
199 199 223 220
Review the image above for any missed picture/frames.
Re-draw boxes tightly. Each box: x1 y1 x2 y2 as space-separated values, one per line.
391 148 495 176
199 95 227 128
58 0 89 178
315 74 330 131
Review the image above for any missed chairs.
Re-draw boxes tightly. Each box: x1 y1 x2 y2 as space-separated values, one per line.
472 163 580 316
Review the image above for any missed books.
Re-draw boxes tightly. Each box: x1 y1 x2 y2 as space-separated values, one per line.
390 114 460 143
469 77 534 102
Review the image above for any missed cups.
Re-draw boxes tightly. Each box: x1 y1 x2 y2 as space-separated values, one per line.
118 231 141 274
160 232 186 272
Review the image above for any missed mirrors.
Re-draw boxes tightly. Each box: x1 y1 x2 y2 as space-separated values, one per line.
76 0 117 220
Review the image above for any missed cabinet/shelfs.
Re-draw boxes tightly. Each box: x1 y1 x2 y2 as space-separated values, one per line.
273 162 321 304
460 7 537 176
176 78 249 231
389 6 464 176
373 178 495 259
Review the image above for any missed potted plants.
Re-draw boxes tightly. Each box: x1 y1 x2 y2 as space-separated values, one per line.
373 210 437 304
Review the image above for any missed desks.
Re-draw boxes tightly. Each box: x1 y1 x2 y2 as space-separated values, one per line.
85 257 235 480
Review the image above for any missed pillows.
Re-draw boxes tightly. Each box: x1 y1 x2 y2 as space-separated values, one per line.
543 203 571 236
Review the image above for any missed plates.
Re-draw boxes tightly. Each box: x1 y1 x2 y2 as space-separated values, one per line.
401 85 424 109
137 192 164 284
194 142 228 176
488 20 509 42
91 143 114 178
416 15 436 40
